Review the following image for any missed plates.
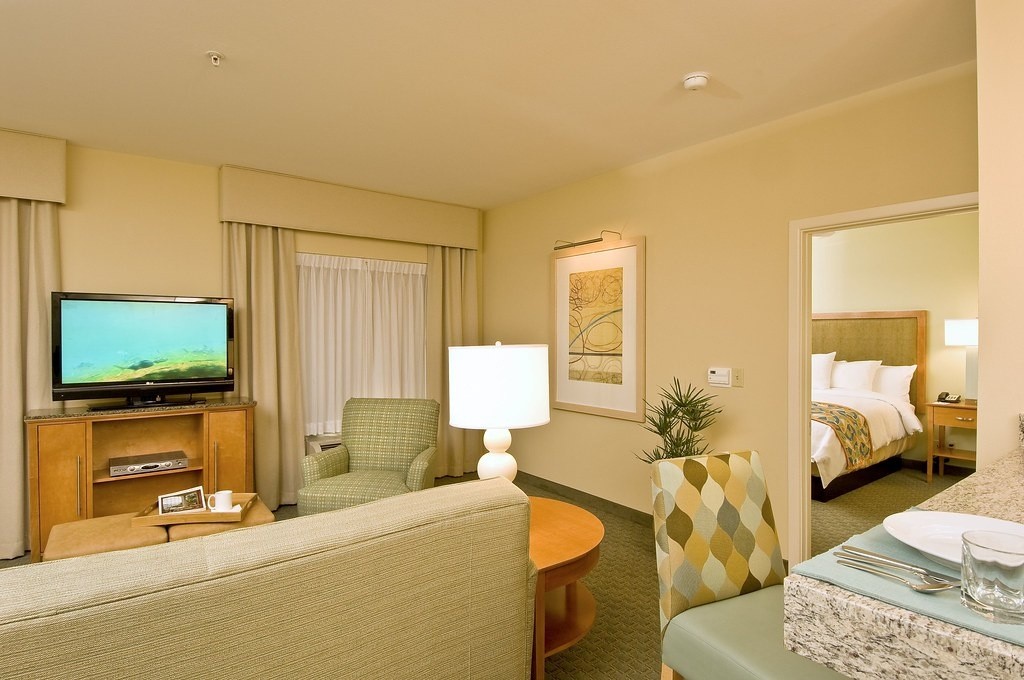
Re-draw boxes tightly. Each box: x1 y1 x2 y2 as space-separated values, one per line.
881 510 1024 573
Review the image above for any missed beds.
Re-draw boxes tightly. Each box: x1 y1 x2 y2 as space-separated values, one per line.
809 386 923 502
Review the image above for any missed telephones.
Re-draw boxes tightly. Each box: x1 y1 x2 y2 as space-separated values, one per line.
937 392 961 403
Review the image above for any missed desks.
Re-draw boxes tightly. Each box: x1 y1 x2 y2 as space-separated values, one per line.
783 437 1024 680
524 496 606 680
40 493 271 563
921 401 977 483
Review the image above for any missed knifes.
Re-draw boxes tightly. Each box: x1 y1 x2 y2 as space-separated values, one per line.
841 544 961 589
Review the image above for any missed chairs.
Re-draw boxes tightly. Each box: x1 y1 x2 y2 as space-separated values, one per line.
648 449 849 680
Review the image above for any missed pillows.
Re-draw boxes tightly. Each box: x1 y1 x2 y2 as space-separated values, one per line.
829 360 882 393
811 351 836 389
873 364 918 402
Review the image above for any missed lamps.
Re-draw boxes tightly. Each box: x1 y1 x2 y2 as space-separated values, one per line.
448 340 550 483
944 317 979 348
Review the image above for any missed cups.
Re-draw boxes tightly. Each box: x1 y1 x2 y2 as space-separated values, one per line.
207 490 233 512
960 530 1024 627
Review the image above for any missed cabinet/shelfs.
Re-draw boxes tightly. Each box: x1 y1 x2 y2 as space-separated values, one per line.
21 397 259 565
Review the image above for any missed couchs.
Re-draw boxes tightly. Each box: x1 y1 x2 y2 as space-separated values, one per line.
296 397 441 516
0 476 536 680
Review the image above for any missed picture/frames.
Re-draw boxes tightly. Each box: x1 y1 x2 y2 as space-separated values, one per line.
550 235 647 422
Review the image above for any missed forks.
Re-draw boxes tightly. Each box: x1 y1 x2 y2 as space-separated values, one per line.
833 551 956 590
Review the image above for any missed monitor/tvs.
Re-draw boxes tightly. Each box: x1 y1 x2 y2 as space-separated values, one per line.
51 292 235 411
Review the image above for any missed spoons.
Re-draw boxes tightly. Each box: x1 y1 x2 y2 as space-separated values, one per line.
837 560 954 594
